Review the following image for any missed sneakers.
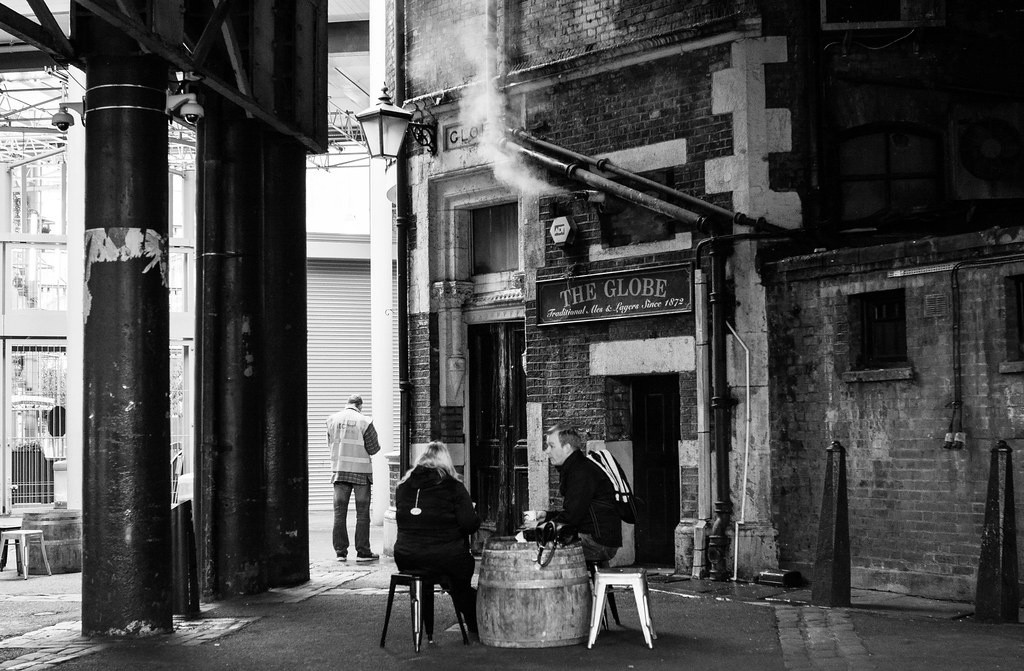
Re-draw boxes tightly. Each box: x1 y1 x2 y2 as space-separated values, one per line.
337 550 347 561
356 552 379 562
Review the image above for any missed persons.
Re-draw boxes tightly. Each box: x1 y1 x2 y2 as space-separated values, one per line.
393 440 477 634
516 424 623 565
325 394 380 561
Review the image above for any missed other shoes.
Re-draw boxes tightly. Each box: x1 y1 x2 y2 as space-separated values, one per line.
468 630 479 641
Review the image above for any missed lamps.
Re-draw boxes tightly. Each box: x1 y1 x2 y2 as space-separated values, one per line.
354 80 438 158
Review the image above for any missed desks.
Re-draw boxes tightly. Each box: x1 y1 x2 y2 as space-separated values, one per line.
19 508 83 575
476 536 591 648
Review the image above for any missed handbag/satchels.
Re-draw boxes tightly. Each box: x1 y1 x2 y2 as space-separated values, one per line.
523 520 580 567
587 449 641 524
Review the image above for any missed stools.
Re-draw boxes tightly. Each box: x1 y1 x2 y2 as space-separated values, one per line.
0 530 53 580
586 566 659 650
376 571 469 652
586 560 622 631
